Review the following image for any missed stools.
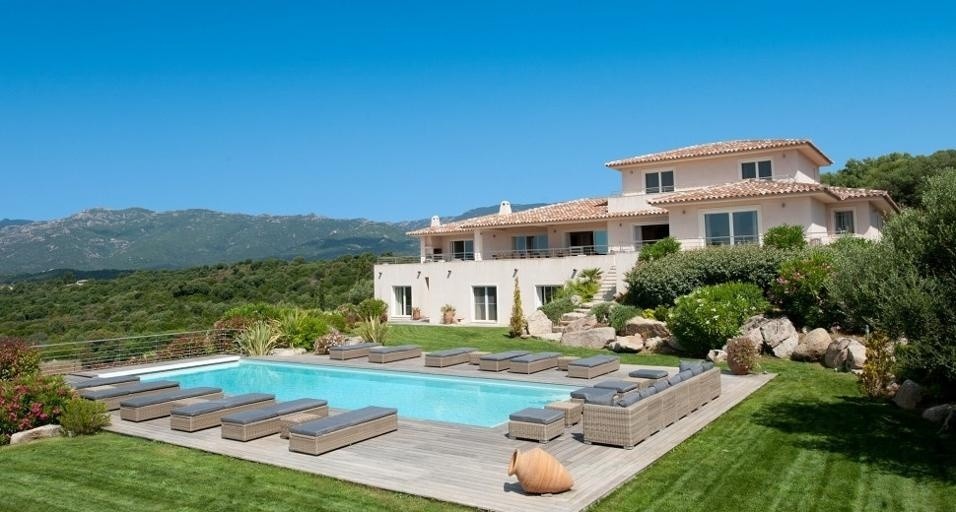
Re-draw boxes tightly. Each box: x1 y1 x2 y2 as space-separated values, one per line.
509 407 565 444
592 378 640 396
620 376 650 391
628 368 668 384
557 356 582 370
570 387 618 403
544 400 582 427
468 351 492 365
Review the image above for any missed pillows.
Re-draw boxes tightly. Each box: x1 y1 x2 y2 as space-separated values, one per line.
617 361 714 408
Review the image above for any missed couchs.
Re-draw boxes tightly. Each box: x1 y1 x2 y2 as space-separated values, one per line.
582 358 721 449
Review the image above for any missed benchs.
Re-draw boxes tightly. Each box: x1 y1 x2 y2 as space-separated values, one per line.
479 351 530 372
368 345 422 364
510 351 563 374
425 348 479 368
329 343 383 360
567 354 621 379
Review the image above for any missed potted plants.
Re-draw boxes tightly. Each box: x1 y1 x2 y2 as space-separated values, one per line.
412 306 421 319
441 304 456 325
